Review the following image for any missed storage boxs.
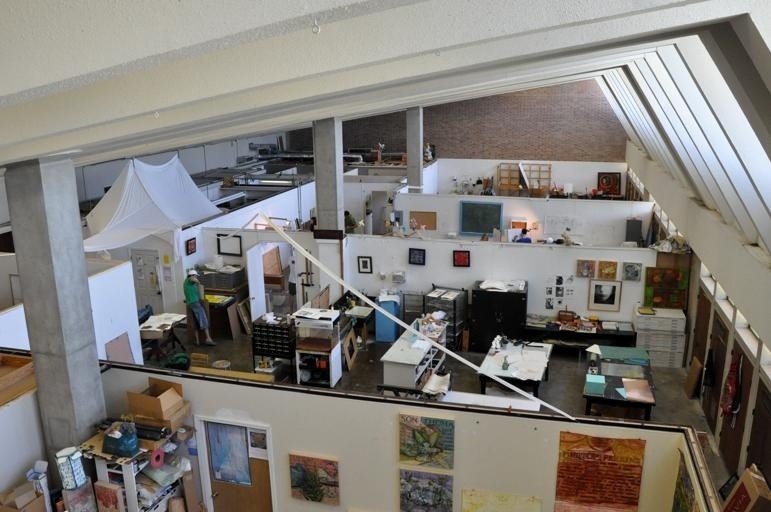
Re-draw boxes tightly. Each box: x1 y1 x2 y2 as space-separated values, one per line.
723 467 771 512
0 489 47 512
126 377 193 432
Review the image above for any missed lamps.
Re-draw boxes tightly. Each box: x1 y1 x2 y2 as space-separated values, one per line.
585 343 602 366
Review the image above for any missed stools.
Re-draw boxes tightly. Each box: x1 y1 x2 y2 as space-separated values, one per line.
212 360 232 371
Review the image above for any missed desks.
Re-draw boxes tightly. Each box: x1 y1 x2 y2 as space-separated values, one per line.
344 303 380 351
139 312 188 361
526 317 636 349
478 340 554 398
583 346 656 421
183 288 249 341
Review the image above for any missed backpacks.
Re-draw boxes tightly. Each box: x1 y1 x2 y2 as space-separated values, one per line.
162 352 190 371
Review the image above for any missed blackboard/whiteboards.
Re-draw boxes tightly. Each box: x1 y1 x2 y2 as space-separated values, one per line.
460 200 503 235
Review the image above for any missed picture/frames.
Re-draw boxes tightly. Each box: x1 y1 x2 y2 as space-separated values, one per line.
186 238 196 255
358 256 372 273
588 279 623 312
453 250 470 267
217 234 242 257
597 172 621 195
409 248 425 265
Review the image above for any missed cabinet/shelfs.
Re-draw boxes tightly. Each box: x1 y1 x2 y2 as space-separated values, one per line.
496 162 551 198
468 281 528 355
94 431 182 512
294 309 343 389
252 315 294 382
423 283 469 352
380 318 449 400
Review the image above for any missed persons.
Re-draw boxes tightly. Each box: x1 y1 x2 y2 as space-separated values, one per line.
309 217 317 231
345 210 358 233
516 228 531 244
183 270 217 346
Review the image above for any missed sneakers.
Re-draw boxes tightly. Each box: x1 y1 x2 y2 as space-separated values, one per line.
205 341 216 346
193 342 200 346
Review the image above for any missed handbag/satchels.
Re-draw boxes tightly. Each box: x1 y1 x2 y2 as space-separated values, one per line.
101 421 139 458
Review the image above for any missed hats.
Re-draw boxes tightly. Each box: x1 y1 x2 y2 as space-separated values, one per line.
188 270 201 276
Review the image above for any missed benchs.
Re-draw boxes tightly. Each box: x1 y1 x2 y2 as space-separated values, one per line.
543 340 588 367
